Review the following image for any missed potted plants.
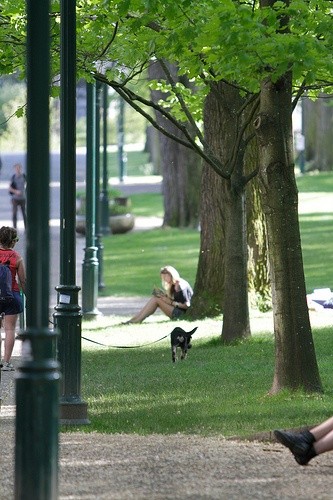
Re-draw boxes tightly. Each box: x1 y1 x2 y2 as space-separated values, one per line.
76 183 134 233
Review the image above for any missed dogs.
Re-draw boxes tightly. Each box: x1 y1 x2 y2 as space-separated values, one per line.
171 326 198 363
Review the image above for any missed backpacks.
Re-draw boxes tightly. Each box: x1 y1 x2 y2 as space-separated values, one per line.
0 260 15 302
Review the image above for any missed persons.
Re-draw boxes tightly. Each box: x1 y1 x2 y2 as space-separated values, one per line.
0 226 26 372
9 163 26 228
274 416 333 465
126 266 193 323
294 130 305 174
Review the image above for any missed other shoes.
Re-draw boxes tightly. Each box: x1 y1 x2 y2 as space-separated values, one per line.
274 429 310 457
294 455 309 465
0 359 15 371
